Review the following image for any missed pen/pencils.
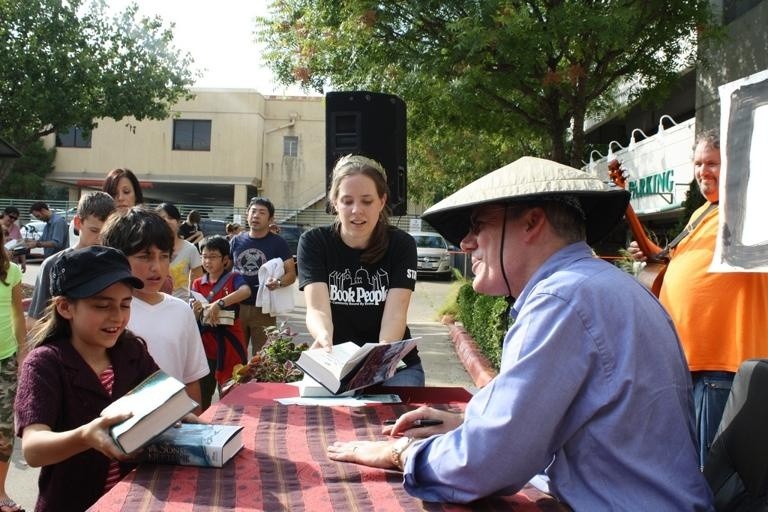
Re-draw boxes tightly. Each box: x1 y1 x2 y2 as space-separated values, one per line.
384 417 444 428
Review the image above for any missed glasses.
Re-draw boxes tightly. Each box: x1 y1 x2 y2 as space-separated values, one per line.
7 214 18 220
200 255 223 261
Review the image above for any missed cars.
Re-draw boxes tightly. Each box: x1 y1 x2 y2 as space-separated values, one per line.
272 224 308 273
19 221 69 261
198 218 228 238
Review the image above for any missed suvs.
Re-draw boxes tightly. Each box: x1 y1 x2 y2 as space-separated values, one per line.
406 231 451 281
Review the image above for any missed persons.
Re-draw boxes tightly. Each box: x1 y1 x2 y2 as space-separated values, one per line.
189 236 252 414
0 206 26 274
268 224 281 234
102 168 144 211
325 155 715 512
14 244 200 512
0 223 27 512
627 127 768 512
177 210 204 256
225 222 241 242
296 154 424 387
228 196 296 356
156 202 204 305
25 190 116 331
98 207 211 418
24 201 70 260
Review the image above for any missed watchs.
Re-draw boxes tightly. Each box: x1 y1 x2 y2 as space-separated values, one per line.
390 435 415 470
216 299 226 310
36 240 42 247
277 278 282 287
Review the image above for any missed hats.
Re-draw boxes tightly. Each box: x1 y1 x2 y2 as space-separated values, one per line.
420 155 631 246
49 247 145 302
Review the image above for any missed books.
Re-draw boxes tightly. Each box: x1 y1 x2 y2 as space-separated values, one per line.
4 239 17 250
134 423 247 468
181 285 236 318
13 246 24 251
202 317 234 326
98 368 200 456
299 371 357 398
295 336 424 397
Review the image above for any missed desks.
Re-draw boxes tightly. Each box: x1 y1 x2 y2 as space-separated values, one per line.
86 385 567 511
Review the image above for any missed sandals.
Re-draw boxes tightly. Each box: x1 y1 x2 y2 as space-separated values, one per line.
0 498 25 512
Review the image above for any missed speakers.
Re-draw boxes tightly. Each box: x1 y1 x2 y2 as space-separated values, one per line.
325 90 408 216
